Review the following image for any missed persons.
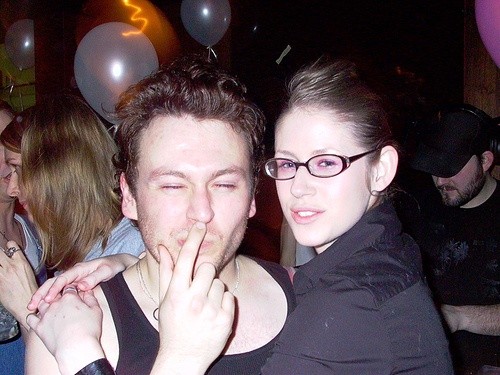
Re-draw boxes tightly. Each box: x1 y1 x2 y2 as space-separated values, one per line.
0 99 49 375
0 92 125 375
409 102 500 375
26 55 453 375
25 49 301 374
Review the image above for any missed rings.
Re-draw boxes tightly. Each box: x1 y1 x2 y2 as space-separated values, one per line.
62 287 77 295
6 246 21 256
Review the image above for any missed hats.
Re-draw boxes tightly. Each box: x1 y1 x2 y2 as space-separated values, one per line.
410 104 495 178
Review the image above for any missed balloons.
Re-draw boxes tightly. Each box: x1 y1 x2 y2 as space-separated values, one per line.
74 0 182 64
0 43 36 112
475 0 500 68
5 19 34 72
73 21 159 125
180 0 232 47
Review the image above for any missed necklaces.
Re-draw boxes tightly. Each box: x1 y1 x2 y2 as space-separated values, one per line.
138 259 241 321
0 219 24 251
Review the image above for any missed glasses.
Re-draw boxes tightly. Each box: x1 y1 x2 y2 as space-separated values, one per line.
265 148 377 180
